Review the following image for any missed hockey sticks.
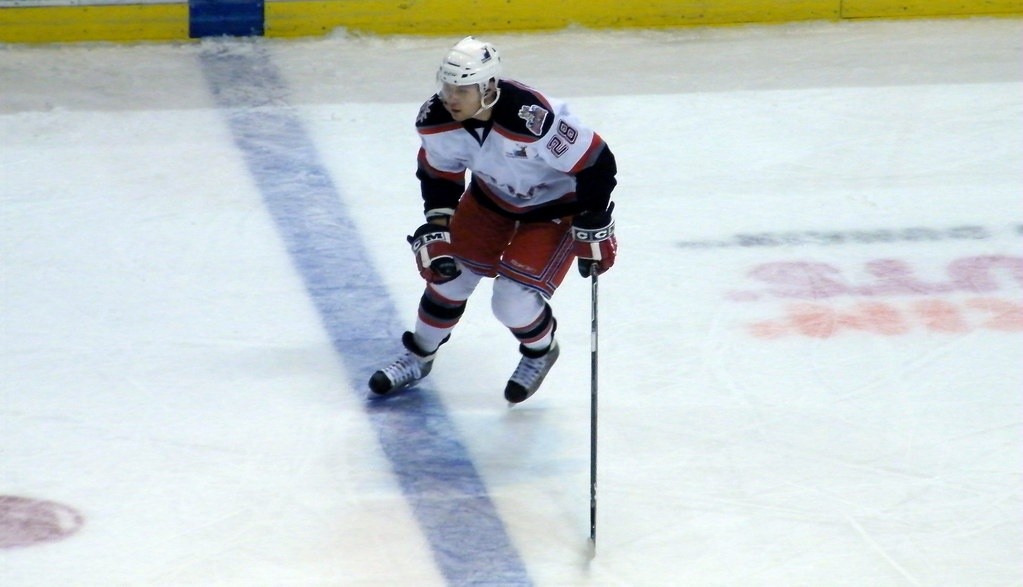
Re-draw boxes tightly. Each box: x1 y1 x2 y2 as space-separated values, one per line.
584 262 605 560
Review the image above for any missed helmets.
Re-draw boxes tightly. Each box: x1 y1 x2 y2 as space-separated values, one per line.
436 36 501 109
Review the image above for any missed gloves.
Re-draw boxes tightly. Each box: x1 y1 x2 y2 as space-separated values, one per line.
407 222 462 284
571 201 617 278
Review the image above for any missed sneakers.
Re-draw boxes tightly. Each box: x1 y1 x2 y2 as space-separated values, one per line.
505 317 559 406
367 331 452 398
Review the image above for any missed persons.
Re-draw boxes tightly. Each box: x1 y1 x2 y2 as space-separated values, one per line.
369 37 618 405
